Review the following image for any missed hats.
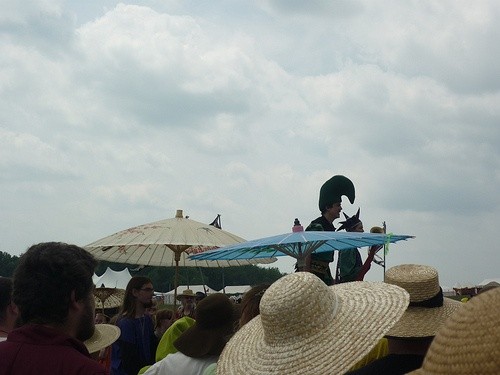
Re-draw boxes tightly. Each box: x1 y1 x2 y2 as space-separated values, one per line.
384 264 466 337
83 323 121 353
337 207 361 232
177 290 198 300
216 272 410 375
420 286 500 375
318 174 355 211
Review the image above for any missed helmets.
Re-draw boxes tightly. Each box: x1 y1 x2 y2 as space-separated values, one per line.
173 293 242 358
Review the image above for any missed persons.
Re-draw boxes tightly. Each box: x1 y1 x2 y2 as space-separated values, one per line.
175 289 196 320
401 286 500 375
195 291 207 305
110 276 159 375
346 264 466 375
237 282 269 328
0 276 24 341
216 272 410 375
295 175 355 287
77 314 121 359
337 207 381 283
143 293 246 375
0 242 110 375
94 311 110 324
154 310 172 337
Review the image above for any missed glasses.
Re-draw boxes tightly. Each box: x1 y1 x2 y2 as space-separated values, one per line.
135 287 155 292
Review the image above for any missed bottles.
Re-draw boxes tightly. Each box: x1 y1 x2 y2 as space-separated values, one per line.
293 218 303 232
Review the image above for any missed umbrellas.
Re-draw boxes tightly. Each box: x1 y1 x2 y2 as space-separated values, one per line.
187 234 416 272
87 288 127 309
83 218 278 316
